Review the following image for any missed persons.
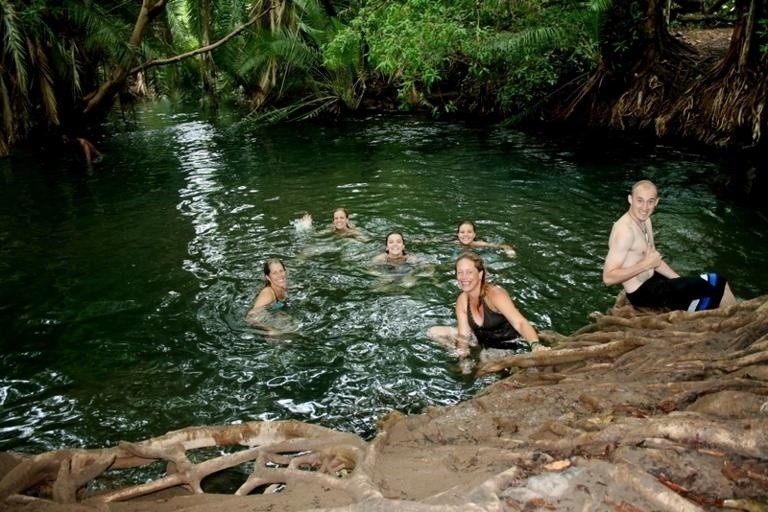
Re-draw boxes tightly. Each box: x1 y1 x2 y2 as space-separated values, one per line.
300 208 367 261
601 178 738 312
426 251 553 375
244 258 299 334
59 129 105 176
411 219 519 259
367 229 434 289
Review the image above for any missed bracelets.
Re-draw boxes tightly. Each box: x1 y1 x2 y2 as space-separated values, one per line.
527 341 542 347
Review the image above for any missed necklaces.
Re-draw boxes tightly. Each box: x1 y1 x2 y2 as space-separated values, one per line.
630 216 649 245
